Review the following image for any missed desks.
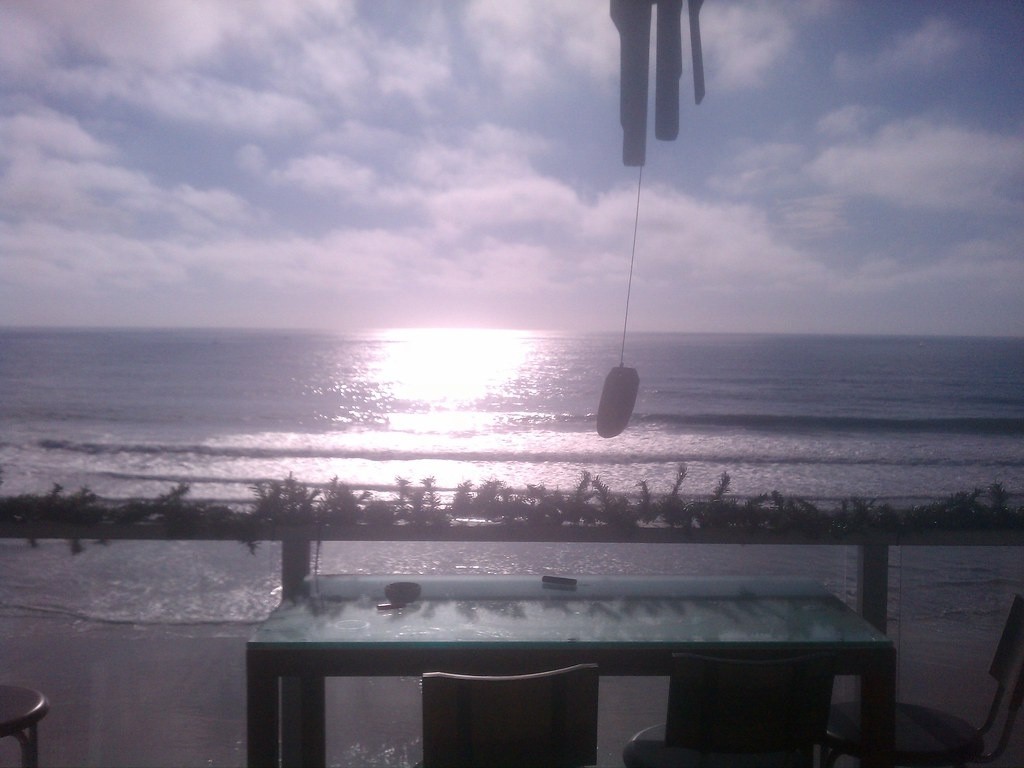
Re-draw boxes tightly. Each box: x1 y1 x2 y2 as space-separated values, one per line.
242 573 897 768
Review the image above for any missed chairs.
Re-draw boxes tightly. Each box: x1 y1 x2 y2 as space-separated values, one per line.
818 593 1024 768
422 662 600 768
0 685 50 768
623 649 838 768
